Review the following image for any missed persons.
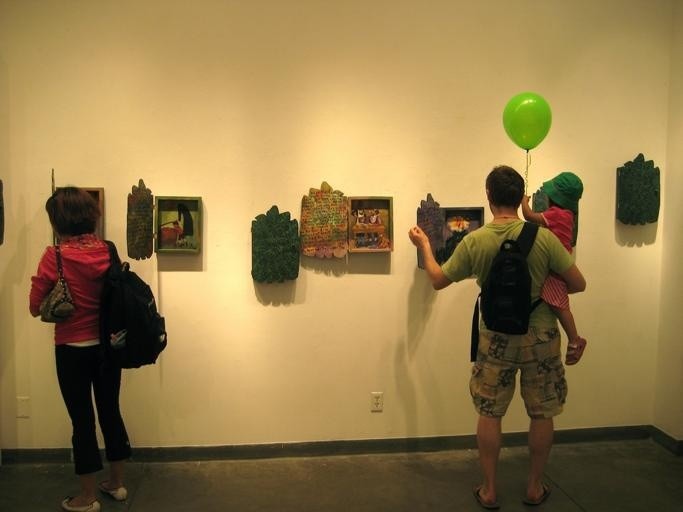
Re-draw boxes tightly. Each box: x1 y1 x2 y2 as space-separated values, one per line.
519 171 587 365
28 184 132 512
409 164 587 509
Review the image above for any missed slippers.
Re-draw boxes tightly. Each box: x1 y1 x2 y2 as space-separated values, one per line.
523 482 551 505
472 485 500 510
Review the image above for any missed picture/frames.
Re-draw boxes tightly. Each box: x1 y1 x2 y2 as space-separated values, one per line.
58 187 104 241
441 208 484 256
155 194 203 255
349 197 393 253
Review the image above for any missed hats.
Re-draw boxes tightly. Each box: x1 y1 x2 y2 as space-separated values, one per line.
543 172 582 211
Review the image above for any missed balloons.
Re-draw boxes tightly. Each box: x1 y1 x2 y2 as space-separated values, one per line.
501 91 554 154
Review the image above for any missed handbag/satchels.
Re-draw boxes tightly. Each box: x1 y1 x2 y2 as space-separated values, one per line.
38 278 75 323
480 239 531 335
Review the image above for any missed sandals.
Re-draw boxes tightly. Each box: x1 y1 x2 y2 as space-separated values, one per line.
565 336 586 365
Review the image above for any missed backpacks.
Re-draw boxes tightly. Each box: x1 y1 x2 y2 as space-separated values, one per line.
100 240 166 369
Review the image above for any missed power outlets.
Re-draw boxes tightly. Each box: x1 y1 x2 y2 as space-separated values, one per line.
372 392 383 411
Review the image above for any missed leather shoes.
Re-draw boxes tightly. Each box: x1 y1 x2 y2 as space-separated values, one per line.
97 481 126 500
61 497 100 512
